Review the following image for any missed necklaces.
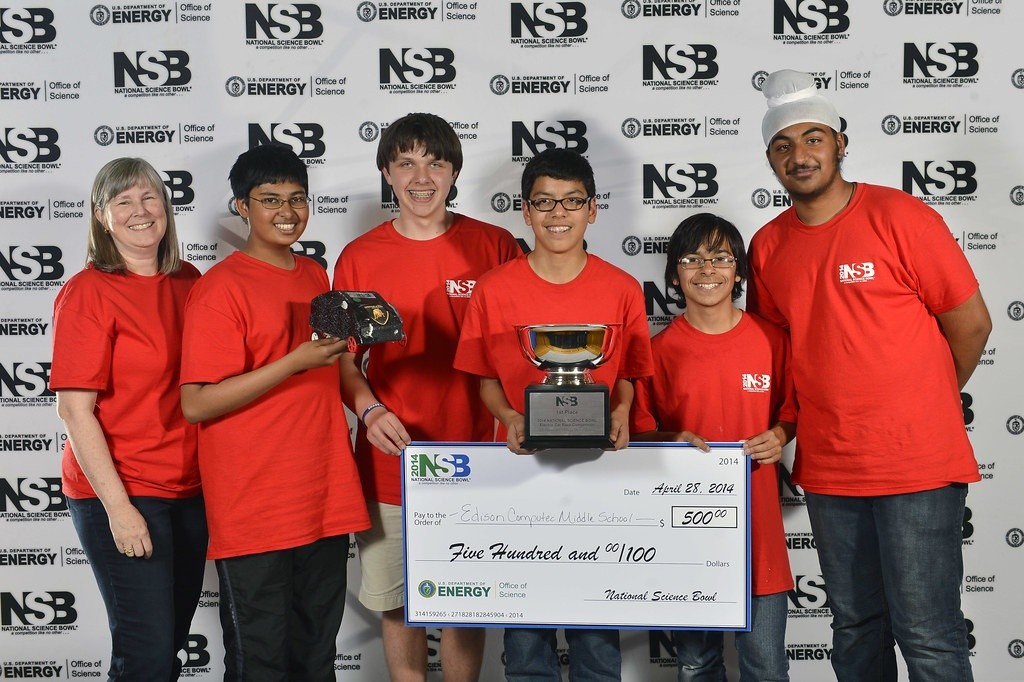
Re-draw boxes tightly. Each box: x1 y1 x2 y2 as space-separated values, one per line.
846 183 854 207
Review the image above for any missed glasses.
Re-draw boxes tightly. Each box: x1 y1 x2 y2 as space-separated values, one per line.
527 197 593 212
245 193 311 209
678 252 737 268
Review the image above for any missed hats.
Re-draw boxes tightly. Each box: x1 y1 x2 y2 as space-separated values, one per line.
759 68 842 148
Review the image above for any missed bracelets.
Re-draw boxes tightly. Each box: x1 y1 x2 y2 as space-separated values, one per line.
362 403 386 429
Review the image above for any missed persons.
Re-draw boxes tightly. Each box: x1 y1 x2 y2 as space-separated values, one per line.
177 142 360 682
50 157 208 682
330 112 525 682
744 68 994 682
453 149 657 682
627 214 797 682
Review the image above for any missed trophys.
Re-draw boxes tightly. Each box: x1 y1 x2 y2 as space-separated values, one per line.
513 322 623 449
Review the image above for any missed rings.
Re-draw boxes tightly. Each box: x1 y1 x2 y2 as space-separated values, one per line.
123 547 133 553
